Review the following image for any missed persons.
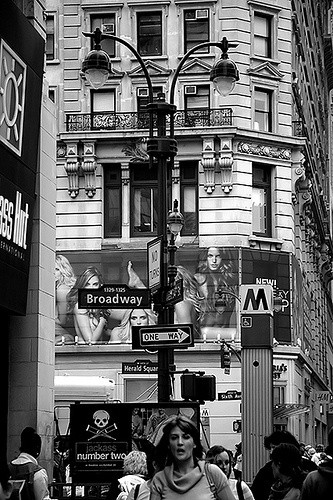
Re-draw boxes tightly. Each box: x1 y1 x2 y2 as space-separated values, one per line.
0 415 333 500
54 247 239 344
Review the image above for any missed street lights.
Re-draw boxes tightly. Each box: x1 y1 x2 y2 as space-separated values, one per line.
83 27 236 395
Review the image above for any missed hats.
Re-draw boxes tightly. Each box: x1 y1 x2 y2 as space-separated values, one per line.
21 427 42 449
